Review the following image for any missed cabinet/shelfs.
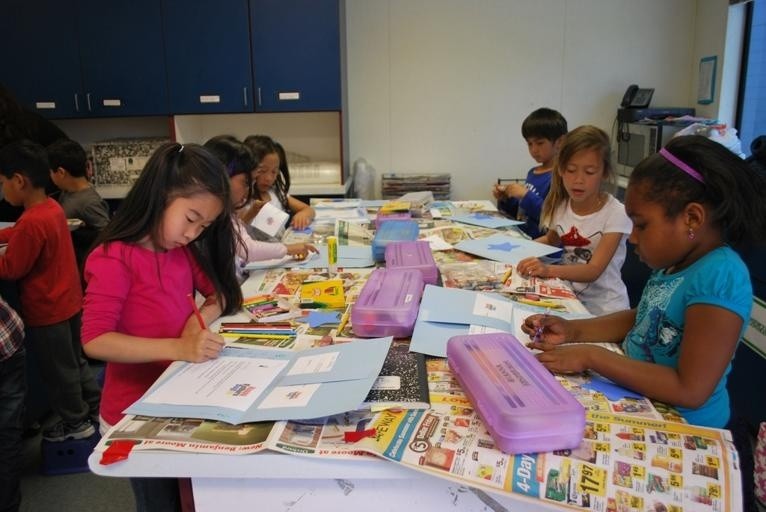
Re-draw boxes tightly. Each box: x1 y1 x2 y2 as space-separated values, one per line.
160 0 347 115
0 0 170 120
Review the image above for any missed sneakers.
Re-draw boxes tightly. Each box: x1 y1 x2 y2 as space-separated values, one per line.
42 402 99 442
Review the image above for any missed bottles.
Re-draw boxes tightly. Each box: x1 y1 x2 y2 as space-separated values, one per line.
326 236 339 272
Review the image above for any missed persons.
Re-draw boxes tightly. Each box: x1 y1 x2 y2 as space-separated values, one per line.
492 107 568 241
518 124 632 316
523 136 766 436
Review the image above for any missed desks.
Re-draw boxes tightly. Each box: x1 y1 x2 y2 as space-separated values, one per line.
85 197 622 512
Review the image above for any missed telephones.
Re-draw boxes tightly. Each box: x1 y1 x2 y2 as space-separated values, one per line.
621 84 655 109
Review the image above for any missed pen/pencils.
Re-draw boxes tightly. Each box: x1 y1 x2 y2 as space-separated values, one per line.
336 313 350 336
241 294 289 318
531 307 552 349
219 322 297 339
501 271 511 283
522 298 567 308
187 294 207 329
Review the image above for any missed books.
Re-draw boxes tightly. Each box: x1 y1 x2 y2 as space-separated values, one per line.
354 345 432 409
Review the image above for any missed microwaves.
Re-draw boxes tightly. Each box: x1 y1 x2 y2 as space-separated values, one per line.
611 117 702 178
